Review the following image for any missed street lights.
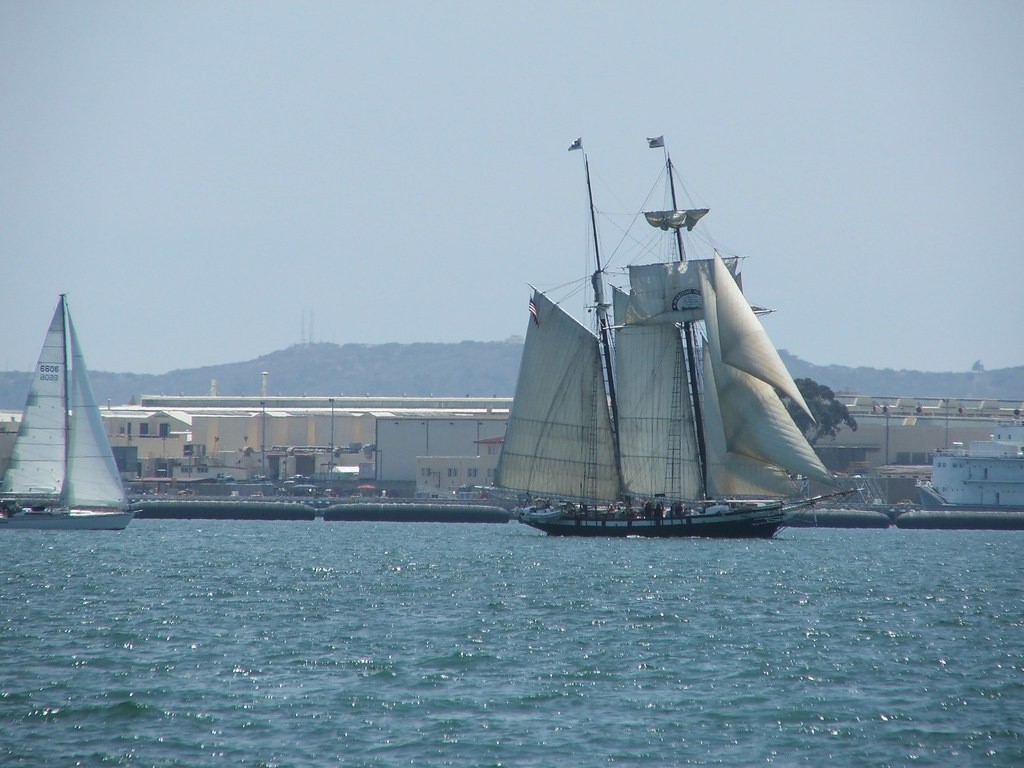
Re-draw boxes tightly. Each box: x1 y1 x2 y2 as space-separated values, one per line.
942 398 950 449
259 401 268 479
328 397 336 480
873 405 890 505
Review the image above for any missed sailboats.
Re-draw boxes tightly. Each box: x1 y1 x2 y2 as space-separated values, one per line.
480 135 868 540
0 293 145 532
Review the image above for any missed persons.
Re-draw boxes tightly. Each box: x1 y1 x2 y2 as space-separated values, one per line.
577 499 712 520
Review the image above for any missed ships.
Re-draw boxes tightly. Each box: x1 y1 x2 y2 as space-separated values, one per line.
932 410 1024 510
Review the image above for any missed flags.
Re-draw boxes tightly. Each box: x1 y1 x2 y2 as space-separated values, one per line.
528 296 540 328
647 136 664 148
568 137 583 151
